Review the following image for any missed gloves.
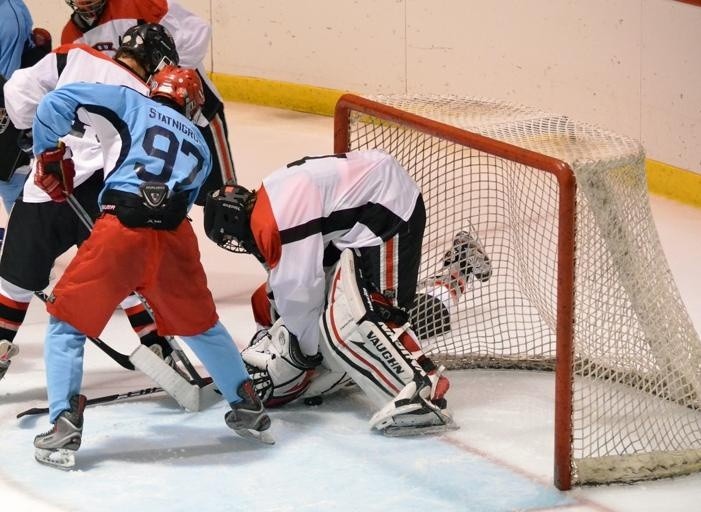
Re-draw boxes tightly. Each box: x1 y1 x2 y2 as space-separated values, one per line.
33 139 77 202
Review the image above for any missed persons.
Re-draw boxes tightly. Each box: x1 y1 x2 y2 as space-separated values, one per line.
203 147 456 434
240 227 493 411
1 0 53 184
30 66 271 453
0 21 211 400
59 1 237 207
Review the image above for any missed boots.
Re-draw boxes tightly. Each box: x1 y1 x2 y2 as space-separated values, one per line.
32 395 88 453
220 378 273 432
443 231 490 281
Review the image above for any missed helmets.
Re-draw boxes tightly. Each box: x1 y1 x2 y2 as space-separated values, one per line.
144 61 207 126
118 22 180 77
65 0 107 21
201 182 259 254
